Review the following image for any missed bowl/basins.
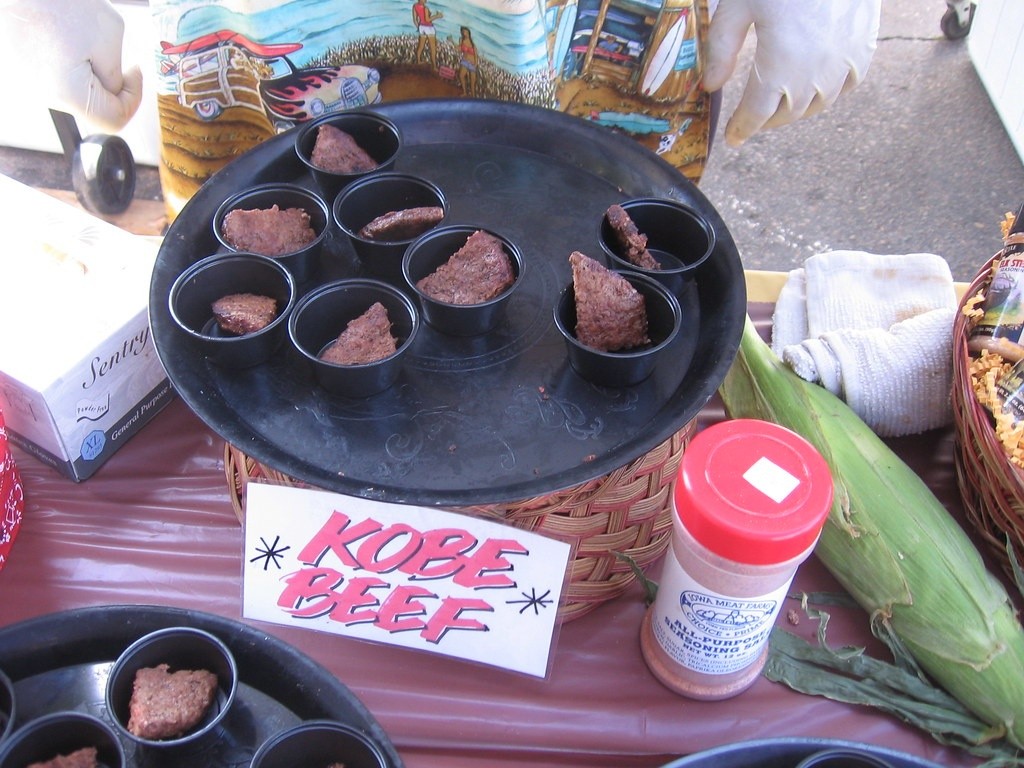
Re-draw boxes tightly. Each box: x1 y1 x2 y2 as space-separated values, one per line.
288 277 420 396
1 712 126 768
105 627 238 757
553 270 683 385
294 111 403 202
213 183 332 285
401 225 526 335
333 171 451 264
167 252 297 368
801 749 892 768
250 720 386 768
599 197 715 299
0 671 17 745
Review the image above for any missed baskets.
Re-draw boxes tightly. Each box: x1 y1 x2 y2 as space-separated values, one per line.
222 415 700 628
951 248 1024 587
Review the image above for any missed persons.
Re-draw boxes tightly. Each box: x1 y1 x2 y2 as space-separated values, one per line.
0 0 880 226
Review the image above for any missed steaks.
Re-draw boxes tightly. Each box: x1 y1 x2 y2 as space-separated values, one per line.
125 662 219 739
206 123 664 368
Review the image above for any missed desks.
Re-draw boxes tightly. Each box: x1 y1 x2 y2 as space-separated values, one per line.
0 267 1024 768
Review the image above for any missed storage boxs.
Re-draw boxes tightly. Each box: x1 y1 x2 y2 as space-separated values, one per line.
1 176 176 485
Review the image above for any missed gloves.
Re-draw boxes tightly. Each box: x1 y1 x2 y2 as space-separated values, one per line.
0 0 143 132
702 0 882 147
